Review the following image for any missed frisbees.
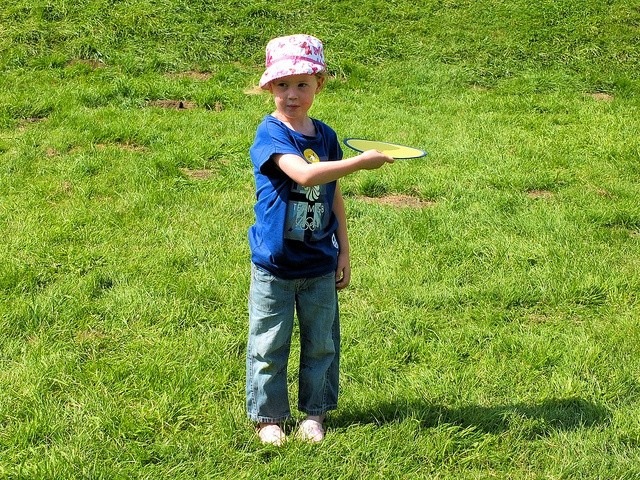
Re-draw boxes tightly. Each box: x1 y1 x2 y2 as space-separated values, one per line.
344 138 428 160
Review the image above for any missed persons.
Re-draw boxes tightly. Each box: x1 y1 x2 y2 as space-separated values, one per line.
243 31 396 448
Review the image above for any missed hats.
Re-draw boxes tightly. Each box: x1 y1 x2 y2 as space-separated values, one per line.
259 34 326 89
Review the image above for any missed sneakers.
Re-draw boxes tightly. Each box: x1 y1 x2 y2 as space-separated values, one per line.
255 425 289 447
295 418 326 443
